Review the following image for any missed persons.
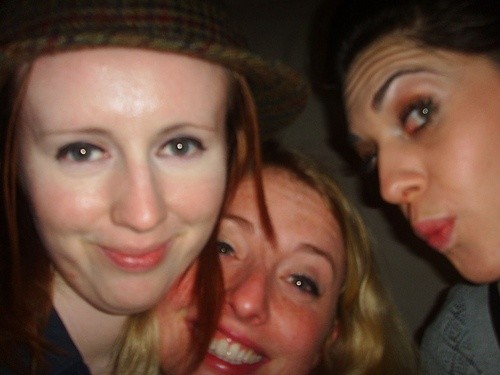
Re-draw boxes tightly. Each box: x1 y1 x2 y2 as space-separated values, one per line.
0 0 500 375
160 138 418 375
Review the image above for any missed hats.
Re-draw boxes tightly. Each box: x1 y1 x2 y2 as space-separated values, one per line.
0 0 307 140
